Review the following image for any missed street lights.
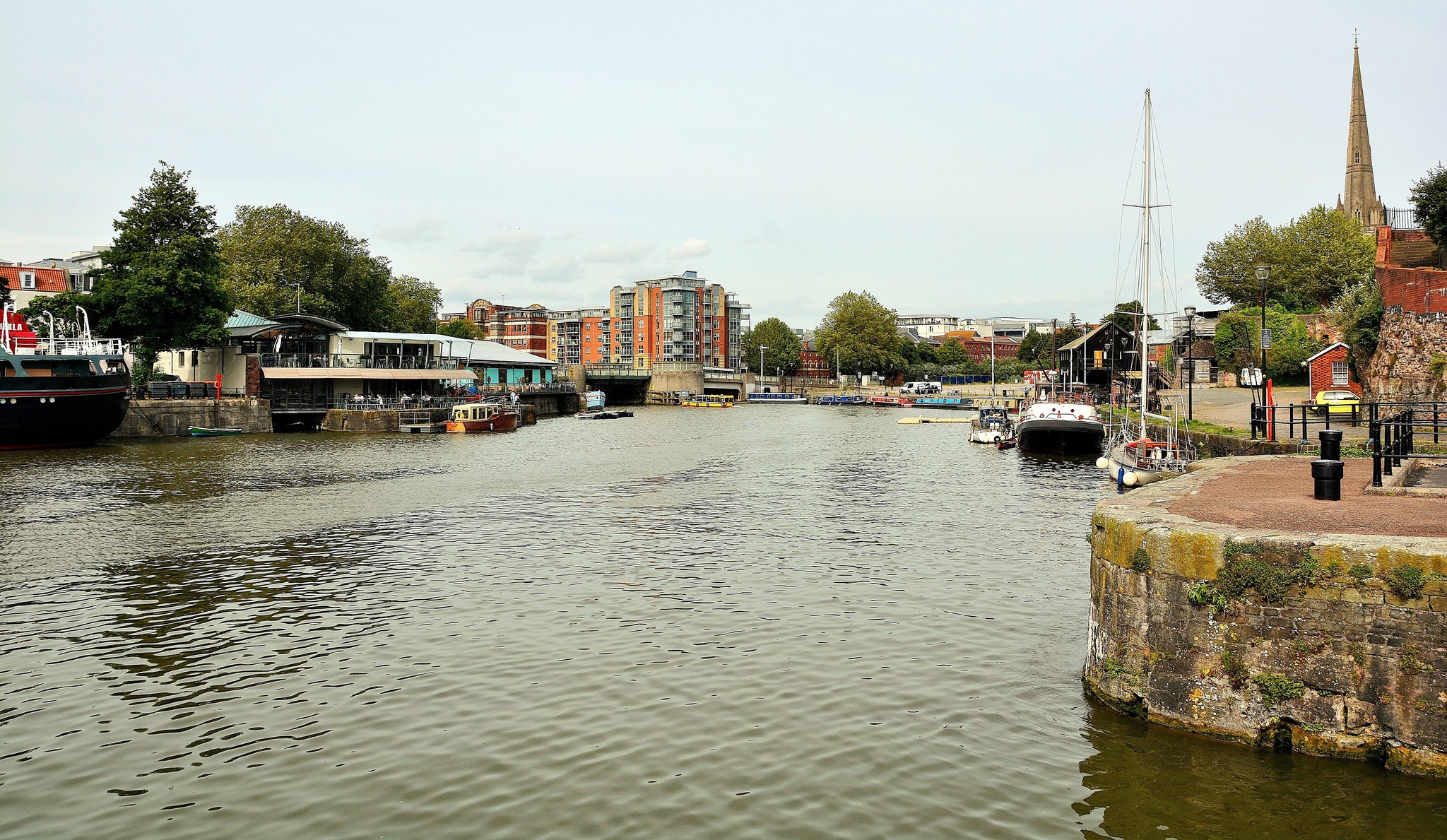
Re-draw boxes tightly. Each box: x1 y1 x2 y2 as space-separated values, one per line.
1121 337 1130 408
777 367 784 376
890 363 894 390
1254 265 1272 437
1105 343 1114 404
1184 306 1196 421
857 361 861 394
790 362 793 393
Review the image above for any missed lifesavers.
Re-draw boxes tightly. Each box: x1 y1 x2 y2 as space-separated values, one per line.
753 389 755 392
456 411 462 418
214 381 222 388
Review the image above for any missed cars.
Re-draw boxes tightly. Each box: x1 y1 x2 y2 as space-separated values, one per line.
1308 390 1363 416
921 386 943 394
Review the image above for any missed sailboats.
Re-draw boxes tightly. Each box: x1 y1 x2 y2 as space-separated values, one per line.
1097 89 1199 485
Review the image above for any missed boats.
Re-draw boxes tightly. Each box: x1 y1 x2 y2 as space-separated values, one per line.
1017 396 1105 455
968 403 1014 443
816 395 1020 412
681 394 734 408
583 390 607 409
0 301 132 451
188 425 243 437
447 402 520 433
749 392 807 404
996 437 1017 449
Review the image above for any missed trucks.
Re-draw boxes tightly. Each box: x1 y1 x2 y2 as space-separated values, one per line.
900 381 926 394
915 383 942 393
